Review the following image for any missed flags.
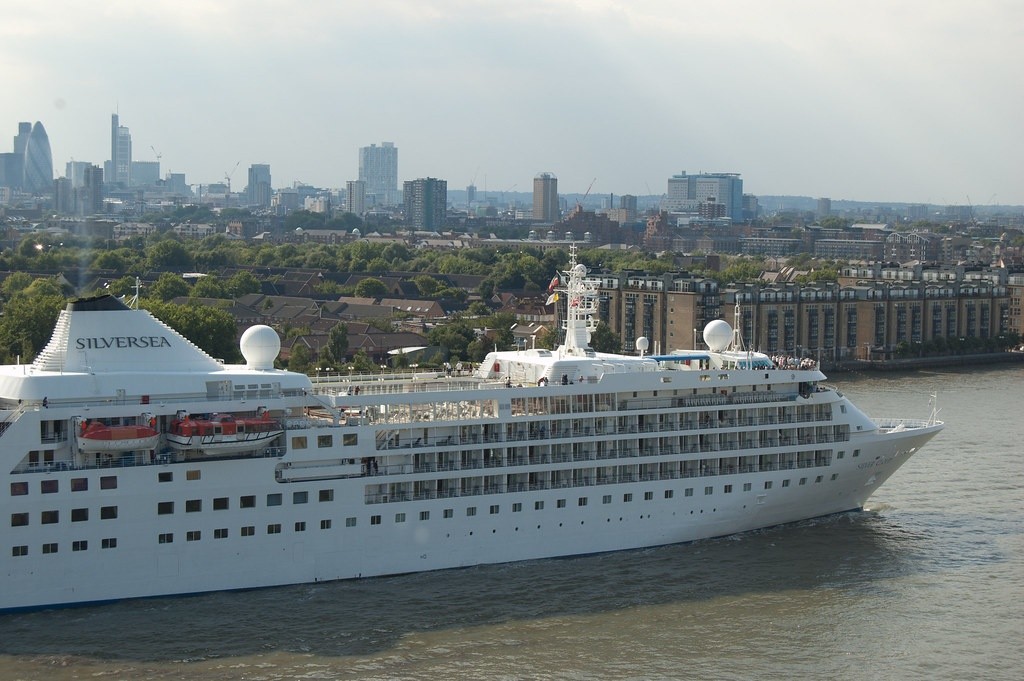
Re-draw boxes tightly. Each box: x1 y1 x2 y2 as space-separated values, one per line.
548 272 559 293
545 291 559 306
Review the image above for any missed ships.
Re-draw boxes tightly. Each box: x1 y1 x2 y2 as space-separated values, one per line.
1 238 948 613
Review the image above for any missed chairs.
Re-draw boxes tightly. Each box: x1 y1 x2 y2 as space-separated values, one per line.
376 400 495 424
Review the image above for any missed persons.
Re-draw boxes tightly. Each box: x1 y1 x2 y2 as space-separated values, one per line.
700 411 739 477
42 396 48 409
365 457 381 476
18 399 24 414
346 360 584 396
100 449 162 467
531 423 549 440
735 353 817 371
